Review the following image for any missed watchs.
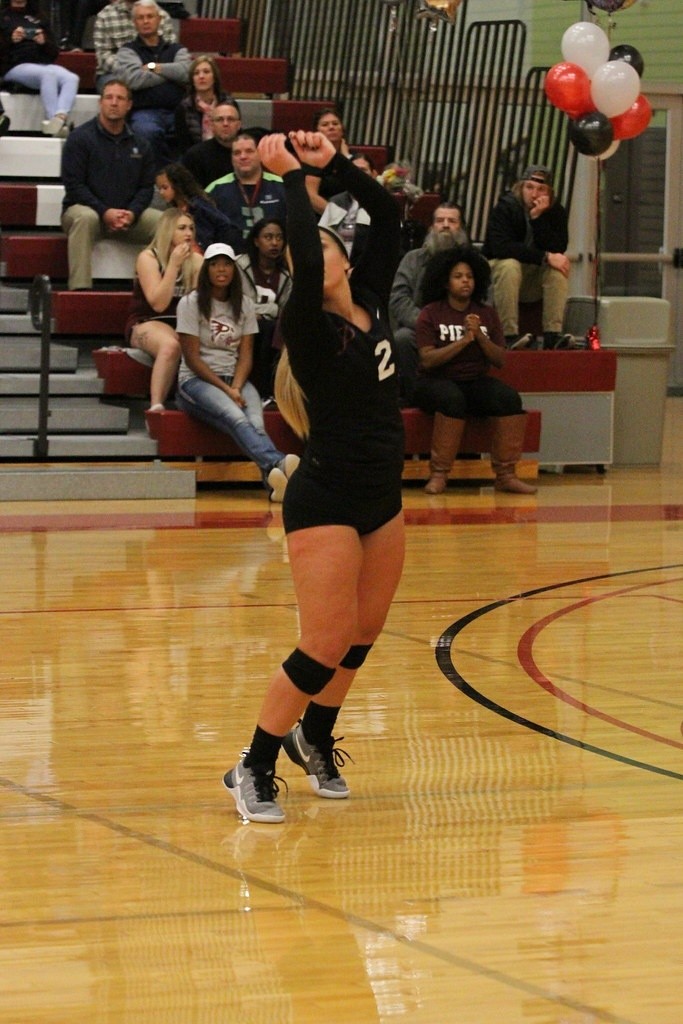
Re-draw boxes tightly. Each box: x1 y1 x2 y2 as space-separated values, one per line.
147 62 156 72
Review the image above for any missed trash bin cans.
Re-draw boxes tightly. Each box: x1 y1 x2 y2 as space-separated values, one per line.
561 297 677 468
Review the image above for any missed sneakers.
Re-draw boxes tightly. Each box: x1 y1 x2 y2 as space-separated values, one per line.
223 751 288 823
293 799 350 843
281 718 355 798
218 816 284 862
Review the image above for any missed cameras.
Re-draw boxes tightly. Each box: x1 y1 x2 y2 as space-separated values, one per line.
24 29 37 40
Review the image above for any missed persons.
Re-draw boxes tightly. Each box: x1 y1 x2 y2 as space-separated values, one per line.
1 0 388 302
390 202 493 348
177 243 300 502
414 249 537 494
480 165 575 350
223 130 405 823
126 210 210 414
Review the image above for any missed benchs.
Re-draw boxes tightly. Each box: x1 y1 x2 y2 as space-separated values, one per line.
0 20 617 477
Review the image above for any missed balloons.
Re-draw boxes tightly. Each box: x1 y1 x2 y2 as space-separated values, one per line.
544 21 652 162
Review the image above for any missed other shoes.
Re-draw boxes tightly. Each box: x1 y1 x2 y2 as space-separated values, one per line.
41 116 64 134
150 403 164 412
543 331 574 350
268 468 288 503
53 126 70 138
278 454 301 479
507 333 533 350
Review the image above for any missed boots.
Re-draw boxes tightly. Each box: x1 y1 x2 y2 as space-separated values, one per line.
425 413 465 494
491 415 538 494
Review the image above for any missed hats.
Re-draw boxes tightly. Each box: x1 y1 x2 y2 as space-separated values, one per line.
318 225 349 257
204 243 237 261
521 165 554 187
